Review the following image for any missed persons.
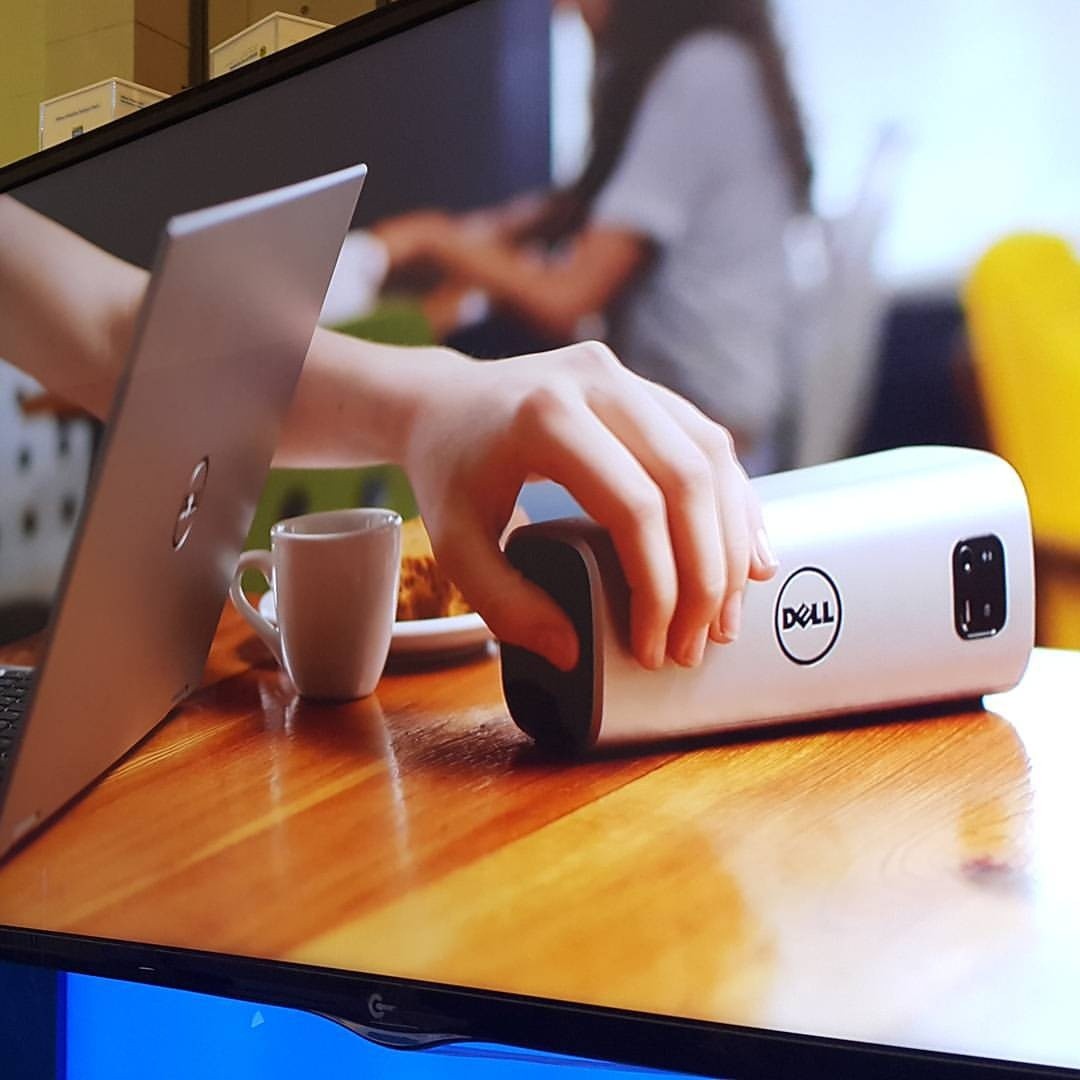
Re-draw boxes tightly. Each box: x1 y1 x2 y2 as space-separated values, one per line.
0 188 778 674
361 2 829 524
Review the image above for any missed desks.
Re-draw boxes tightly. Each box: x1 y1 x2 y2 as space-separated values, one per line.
0 579 1080 1079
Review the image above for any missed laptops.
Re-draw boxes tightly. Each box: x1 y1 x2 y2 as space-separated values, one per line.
1 165 374 857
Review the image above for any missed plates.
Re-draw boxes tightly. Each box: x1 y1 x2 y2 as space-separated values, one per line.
257 586 493 663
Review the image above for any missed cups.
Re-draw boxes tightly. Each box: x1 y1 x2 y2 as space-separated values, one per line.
229 506 400 702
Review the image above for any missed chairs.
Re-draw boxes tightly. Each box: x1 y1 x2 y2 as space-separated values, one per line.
962 234 1080 655
788 118 913 469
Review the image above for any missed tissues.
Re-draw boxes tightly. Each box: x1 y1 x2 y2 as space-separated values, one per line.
240 227 448 597
